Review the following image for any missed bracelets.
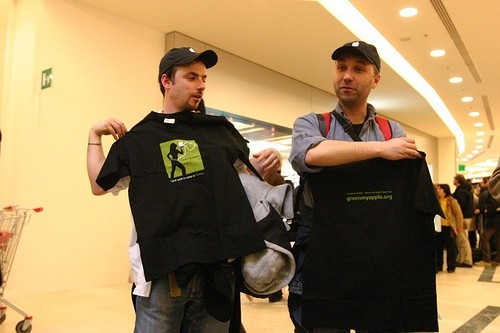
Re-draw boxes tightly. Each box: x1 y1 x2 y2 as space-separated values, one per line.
88 143 102 145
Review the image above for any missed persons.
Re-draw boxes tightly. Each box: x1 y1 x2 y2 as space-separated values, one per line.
287 38 428 333
435 156 500 273
86 46 283 333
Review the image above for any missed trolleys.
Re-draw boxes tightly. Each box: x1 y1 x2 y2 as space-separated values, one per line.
0 206 43 332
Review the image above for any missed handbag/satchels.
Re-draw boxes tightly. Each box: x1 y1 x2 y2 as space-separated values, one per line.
456 219 468 232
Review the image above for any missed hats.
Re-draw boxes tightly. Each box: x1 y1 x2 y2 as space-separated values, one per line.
332 40 380 71
158 46 218 77
